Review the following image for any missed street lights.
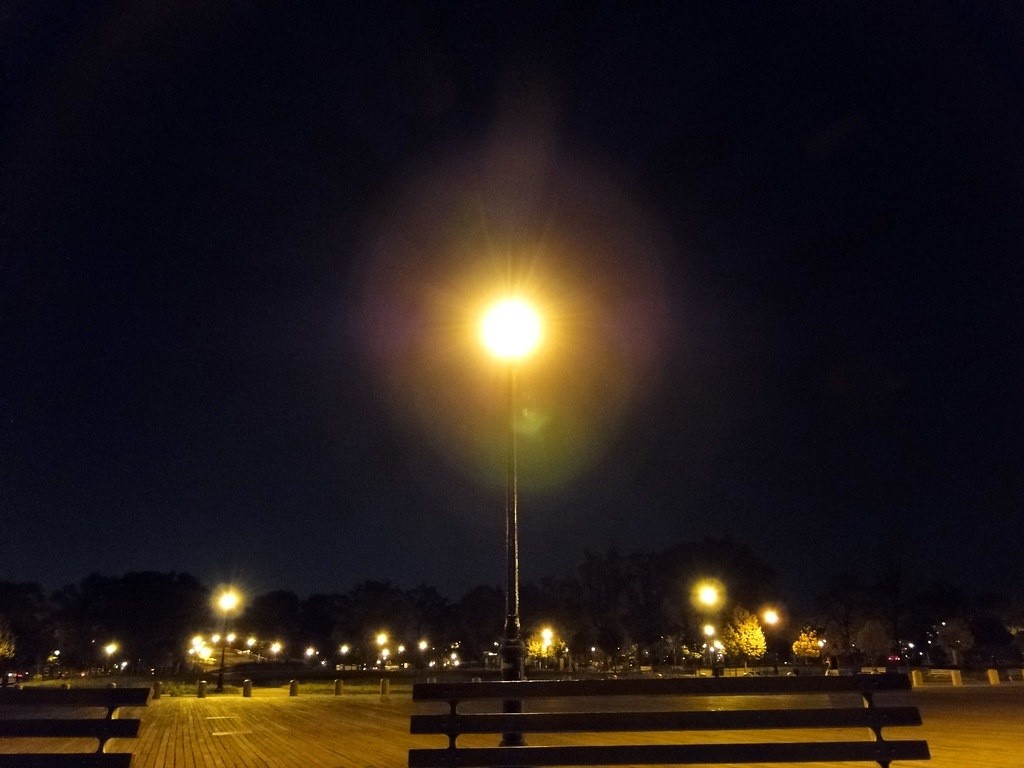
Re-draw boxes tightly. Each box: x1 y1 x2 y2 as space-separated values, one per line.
474 289 547 746
214 590 236 693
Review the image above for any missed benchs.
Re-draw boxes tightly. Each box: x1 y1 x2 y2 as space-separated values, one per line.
410 671 931 768
0 682 155 768
